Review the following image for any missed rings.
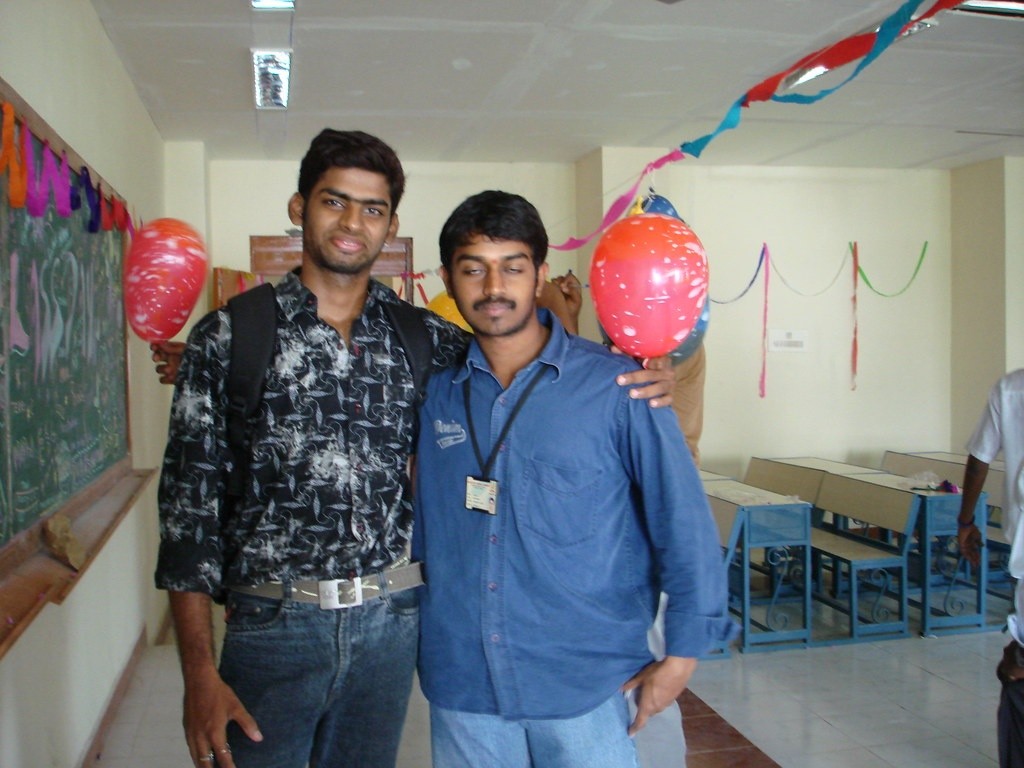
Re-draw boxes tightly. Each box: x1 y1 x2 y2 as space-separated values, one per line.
215 743 232 755
200 751 215 762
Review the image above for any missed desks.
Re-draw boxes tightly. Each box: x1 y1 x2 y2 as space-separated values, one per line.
695 450 1019 660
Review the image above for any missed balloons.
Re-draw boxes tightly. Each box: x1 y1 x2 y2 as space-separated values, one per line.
424 290 475 339
591 194 710 368
121 219 208 347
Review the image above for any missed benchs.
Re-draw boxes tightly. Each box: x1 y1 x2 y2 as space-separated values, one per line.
705 492 746 653
864 451 977 592
764 472 922 637
981 465 1015 602
735 457 830 598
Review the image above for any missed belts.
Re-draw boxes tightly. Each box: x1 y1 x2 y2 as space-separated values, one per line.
230 557 427 609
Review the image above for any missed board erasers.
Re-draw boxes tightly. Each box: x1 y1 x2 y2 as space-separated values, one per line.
42 515 87 572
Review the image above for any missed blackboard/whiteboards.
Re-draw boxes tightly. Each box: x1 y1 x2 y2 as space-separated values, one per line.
1 101 129 547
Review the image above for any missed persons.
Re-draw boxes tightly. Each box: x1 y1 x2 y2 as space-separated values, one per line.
958 369 1024 768
488 498 495 513
156 129 677 768
149 187 732 768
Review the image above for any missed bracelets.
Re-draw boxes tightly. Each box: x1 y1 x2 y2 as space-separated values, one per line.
958 515 975 528
